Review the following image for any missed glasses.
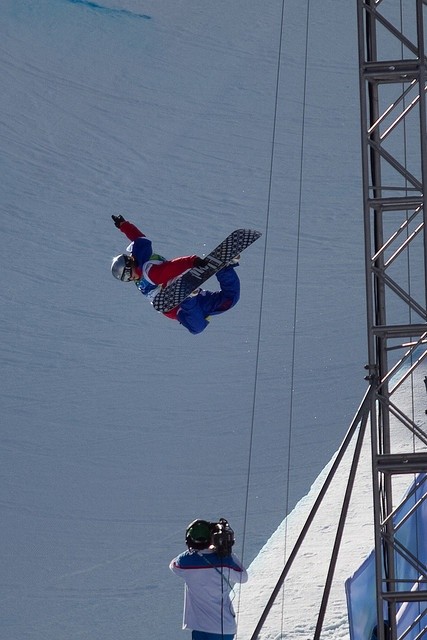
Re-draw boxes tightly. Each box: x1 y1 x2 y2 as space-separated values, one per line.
120 255 132 282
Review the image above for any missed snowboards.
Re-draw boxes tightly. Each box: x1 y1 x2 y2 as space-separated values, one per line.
151 228 262 313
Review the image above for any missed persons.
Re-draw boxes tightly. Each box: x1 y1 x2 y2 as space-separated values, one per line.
110 213 242 336
169 517 247 639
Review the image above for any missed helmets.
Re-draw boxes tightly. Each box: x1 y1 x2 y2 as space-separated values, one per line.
184 518 213 552
111 254 136 282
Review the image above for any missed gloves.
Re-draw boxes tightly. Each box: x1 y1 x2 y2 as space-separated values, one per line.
111 214 126 229
192 256 208 269
